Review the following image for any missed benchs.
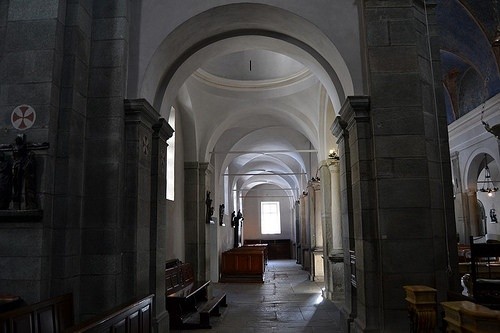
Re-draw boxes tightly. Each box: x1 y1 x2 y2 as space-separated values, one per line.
165 262 212 328
236 238 291 265
199 292 228 329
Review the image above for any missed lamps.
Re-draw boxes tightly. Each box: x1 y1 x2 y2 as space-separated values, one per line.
479 153 498 197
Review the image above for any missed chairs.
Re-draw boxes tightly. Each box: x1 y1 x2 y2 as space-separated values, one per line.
469 236 500 312
